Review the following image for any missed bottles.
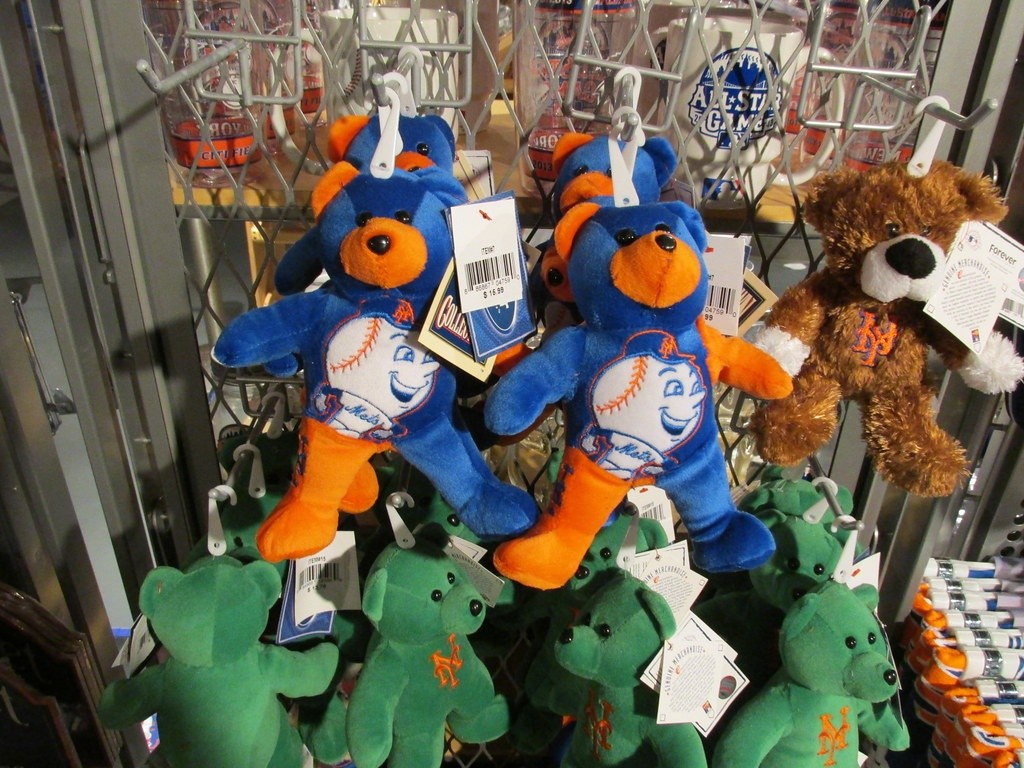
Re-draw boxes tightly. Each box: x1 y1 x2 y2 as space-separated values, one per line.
512 0 926 200
141 0 498 188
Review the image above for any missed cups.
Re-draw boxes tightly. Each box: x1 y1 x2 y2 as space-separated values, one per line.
633 0 794 140
658 19 844 210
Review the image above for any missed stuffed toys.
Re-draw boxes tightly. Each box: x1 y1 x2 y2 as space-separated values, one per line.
95 112 1009 768
